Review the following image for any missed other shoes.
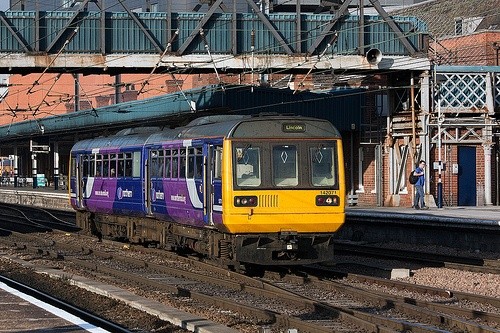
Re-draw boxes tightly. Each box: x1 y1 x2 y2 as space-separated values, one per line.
413 206 420 209
421 206 429 210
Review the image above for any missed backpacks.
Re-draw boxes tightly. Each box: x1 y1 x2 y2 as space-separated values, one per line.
408 169 419 184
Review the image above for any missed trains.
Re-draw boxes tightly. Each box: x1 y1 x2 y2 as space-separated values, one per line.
68 113 348 267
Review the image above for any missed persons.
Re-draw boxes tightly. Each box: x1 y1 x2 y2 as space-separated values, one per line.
412 160 429 210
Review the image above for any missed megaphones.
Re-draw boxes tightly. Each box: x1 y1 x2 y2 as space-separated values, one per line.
366 48 383 65
